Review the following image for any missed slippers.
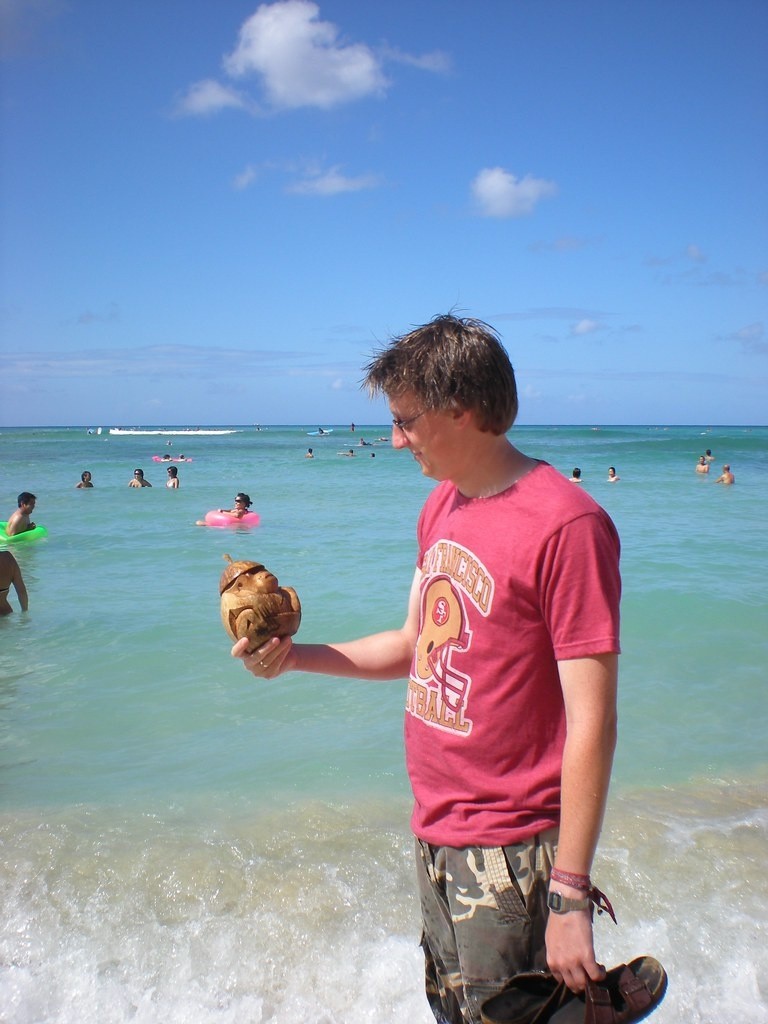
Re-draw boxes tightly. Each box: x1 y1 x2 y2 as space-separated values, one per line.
478 955 668 1024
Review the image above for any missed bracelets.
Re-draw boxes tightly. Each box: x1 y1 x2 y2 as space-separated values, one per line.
550 868 618 925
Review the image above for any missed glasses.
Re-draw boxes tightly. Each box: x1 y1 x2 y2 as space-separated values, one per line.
134 473 140 475
234 500 241 503
392 401 441 432
168 471 171 473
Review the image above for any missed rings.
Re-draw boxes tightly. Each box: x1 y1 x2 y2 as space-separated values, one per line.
260 661 269 668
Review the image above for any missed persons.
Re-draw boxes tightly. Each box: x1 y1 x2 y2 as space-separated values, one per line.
607 467 621 483
0 551 28 617
219 316 622 1024
75 471 94 489
715 464 736 485
696 456 709 474
304 422 389 459
705 449 715 462
7 492 37 537
568 468 582 483
218 492 253 520
128 468 152 488
166 466 179 489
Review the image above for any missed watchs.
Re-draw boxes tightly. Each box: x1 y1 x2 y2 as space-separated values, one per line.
547 890 589 915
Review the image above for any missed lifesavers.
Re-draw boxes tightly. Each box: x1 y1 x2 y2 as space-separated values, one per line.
153 456 192 462
205 510 260 528
0 521 47 545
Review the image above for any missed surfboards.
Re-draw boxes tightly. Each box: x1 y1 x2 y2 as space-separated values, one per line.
307 429 333 435
